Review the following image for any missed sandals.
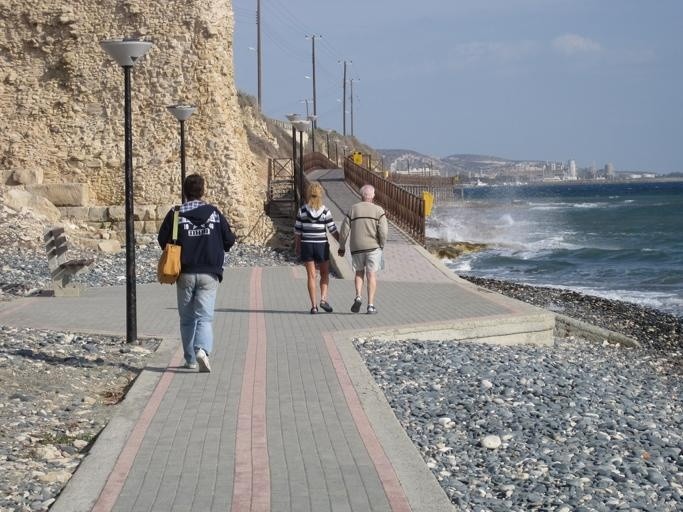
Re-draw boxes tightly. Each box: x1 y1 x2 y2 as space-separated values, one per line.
311 299 333 314
351 296 362 312
367 305 377 314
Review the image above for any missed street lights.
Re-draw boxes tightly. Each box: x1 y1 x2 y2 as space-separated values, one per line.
165 104 199 203
324 129 333 160
97 37 155 341
341 144 348 160
333 139 339 165
308 114 318 153
284 113 302 207
290 119 311 210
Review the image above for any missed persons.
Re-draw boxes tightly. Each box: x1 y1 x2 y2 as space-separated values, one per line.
294 183 340 314
157 173 235 374
338 183 388 315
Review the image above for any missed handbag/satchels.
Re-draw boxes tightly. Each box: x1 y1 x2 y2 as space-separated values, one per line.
157 242 182 284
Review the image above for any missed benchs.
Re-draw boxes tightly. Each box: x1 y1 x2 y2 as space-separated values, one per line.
44 227 94 297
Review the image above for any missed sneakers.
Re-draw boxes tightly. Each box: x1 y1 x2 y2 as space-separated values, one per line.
184 360 196 369
196 349 211 372
